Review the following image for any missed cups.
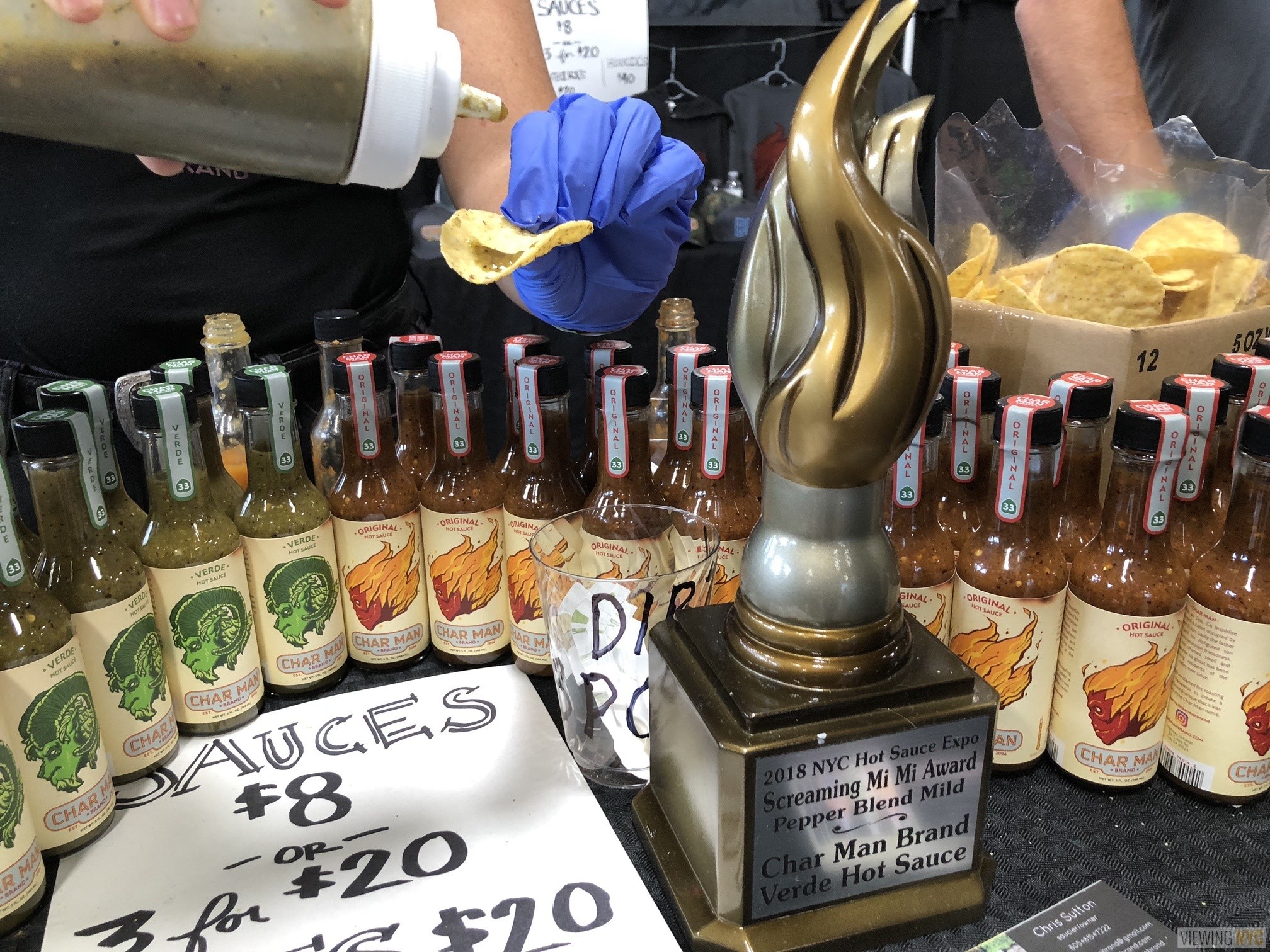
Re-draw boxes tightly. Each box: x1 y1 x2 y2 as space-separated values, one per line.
529 503 721 787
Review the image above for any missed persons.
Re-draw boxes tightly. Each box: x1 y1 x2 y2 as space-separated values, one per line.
910 0 1269 261
0 0 705 388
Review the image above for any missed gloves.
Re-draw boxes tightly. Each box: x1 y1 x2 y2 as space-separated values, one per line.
1106 207 1182 251
501 93 704 332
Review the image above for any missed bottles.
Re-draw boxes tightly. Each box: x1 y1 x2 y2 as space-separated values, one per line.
2 0 507 190
723 171 743 200
2 296 1270 938
704 179 724 201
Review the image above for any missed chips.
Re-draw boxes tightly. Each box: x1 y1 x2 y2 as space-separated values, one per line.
440 209 594 285
946 210 1270 327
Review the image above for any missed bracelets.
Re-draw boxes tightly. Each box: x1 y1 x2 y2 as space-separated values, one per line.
1088 192 1190 251
